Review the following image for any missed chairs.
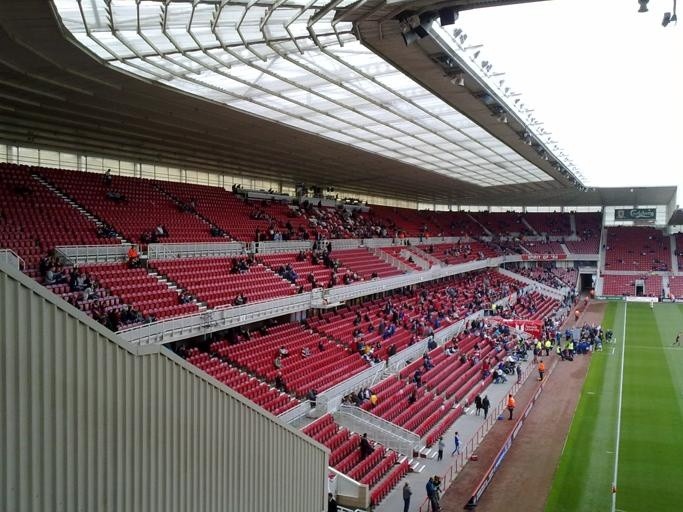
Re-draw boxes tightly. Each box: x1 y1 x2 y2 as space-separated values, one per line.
0 164 602 511
602 225 682 301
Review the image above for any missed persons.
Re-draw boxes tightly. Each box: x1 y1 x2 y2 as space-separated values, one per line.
368 445 374 455
432 475 442 502
616 231 682 313
451 431 460 456
33 168 616 422
358 433 370 460
327 493 339 512
671 332 681 347
436 437 445 461
425 477 442 512
402 482 413 512
